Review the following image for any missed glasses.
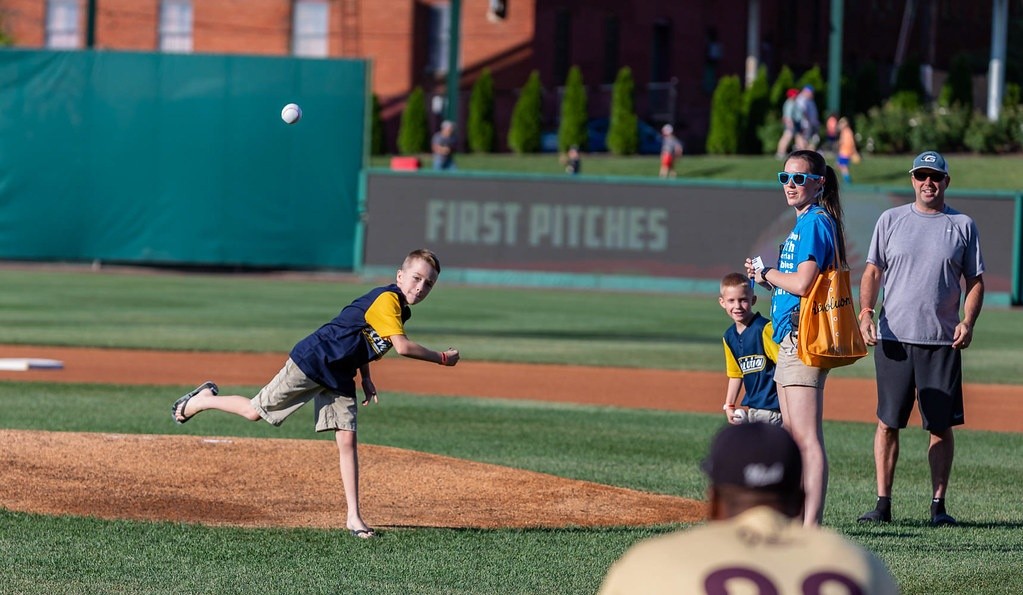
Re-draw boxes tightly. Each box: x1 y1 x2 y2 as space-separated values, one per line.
911 173 948 182
778 172 821 186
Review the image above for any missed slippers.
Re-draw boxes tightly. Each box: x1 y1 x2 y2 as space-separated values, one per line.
170 381 219 424
349 528 374 539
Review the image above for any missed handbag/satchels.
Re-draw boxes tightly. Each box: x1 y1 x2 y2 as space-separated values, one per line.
796 211 869 369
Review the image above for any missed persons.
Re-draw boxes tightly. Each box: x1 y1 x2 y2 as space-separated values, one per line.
171 248 460 539
774 84 861 183
556 144 582 175
744 150 848 526
855 150 986 528
431 83 457 172
658 123 683 181
596 419 901 595
718 272 783 426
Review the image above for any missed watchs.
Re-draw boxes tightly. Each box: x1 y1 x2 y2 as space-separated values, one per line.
761 266 774 280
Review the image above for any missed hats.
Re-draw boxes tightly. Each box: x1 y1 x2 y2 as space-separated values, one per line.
908 151 948 173
700 421 805 496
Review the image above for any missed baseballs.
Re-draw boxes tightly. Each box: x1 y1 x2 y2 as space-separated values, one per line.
732 409 748 426
282 103 302 125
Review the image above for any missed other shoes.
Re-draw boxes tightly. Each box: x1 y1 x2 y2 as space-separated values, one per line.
931 513 956 527
857 509 892 522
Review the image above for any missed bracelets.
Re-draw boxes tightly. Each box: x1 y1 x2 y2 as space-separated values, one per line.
723 404 735 409
438 351 448 365
858 307 875 321
